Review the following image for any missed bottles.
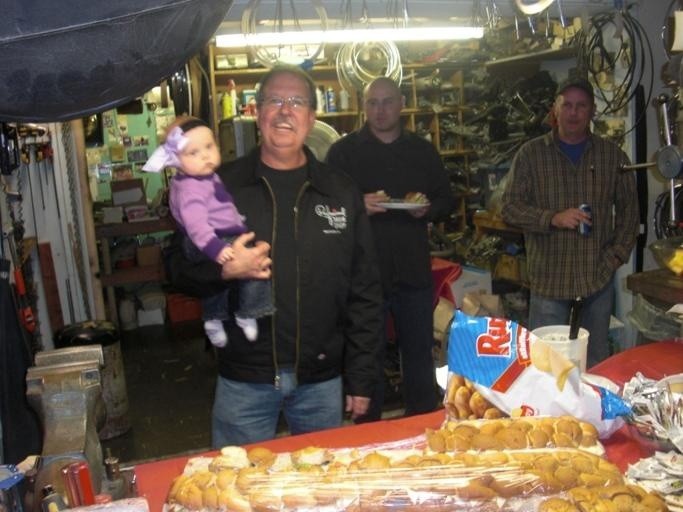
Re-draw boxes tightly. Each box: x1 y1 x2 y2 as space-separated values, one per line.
250 99 258 116
222 92 232 120
315 86 323 114
325 85 338 112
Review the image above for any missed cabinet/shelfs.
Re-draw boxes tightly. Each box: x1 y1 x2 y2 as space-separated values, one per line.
96 219 208 367
472 211 529 317
208 38 478 259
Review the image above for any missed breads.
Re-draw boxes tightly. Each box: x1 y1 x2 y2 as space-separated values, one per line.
423 414 599 452
163 445 670 511
443 372 503 422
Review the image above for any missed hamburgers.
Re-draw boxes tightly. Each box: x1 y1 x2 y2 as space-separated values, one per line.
404 191 428 204
376 189 391 202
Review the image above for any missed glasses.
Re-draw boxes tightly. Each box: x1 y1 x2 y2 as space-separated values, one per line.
262 96 310 110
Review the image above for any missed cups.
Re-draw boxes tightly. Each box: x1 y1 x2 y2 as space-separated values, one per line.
531 323 589 374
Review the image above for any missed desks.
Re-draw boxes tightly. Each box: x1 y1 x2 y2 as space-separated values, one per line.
627 266 683 346
117 337 683 512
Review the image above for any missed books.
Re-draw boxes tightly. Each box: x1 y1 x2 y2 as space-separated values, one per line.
94 205 161 224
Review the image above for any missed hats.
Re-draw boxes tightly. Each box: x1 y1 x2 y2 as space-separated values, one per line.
556 78 593 96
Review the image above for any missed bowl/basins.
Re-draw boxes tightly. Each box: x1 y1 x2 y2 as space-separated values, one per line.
649 235 683 279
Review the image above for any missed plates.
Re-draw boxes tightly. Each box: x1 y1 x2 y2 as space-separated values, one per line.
373 199 431 210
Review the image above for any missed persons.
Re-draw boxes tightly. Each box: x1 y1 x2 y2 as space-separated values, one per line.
324 76 456 424
500 77 641 369
156 65 387 452
141 115 277 348
